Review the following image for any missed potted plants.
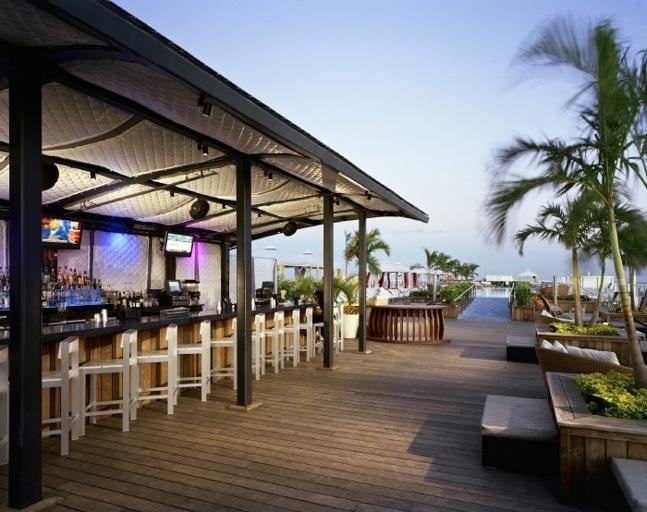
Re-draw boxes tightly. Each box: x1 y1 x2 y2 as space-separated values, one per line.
332 281 364 339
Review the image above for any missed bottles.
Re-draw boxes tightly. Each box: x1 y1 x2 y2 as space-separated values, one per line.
0 266 10 307
41 265 159 307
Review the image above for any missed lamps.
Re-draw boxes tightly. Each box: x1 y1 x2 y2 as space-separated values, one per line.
222 204 225 209
198 143 208 156
170 191 174 198
258 213 261 217
364 191 372 200
264 171 272 179
198 97 212 118
91 172 96 181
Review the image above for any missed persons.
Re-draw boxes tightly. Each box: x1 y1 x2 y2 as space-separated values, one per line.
41 219 81 244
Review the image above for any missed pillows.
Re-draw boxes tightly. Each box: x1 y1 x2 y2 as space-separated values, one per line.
541 339 621 366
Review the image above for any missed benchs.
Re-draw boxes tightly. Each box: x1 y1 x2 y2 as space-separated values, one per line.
505 335 537 363
479 394 559 468
610 457 647 512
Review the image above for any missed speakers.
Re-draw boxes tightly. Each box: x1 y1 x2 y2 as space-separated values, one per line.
189 199 210 220
41 161 59 191
282 222 297 237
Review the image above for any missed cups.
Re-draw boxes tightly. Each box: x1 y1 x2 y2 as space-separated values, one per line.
94 308 108 323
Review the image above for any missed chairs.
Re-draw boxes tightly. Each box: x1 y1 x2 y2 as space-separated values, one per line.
535 343 634 399
539 294 571 324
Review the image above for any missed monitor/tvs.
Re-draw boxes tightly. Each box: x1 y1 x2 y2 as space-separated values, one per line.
162 231 194 257
40 214 84 250
165 280 182 293
